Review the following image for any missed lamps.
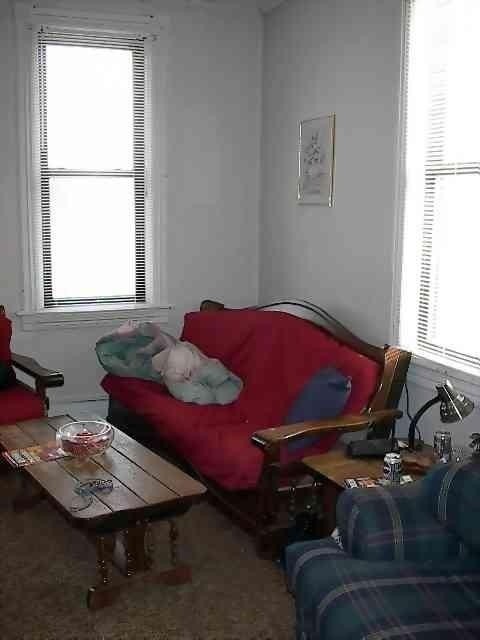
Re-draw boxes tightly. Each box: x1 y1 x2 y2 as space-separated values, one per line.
396 379 475 472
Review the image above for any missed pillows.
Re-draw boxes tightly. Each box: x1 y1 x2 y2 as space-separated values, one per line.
283 367 351 428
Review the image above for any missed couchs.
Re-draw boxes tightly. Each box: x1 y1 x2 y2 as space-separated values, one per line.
100 299 412 551
1 306 64 415
285 455 479 639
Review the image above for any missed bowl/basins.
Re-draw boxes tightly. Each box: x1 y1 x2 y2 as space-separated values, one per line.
54 421 114 458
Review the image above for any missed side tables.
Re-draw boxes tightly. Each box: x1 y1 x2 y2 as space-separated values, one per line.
300 437 464 538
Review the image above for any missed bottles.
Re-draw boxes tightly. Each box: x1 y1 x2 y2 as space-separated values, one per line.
383 453 401 483
433 431 453 464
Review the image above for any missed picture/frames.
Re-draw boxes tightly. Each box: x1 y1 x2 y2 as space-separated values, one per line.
297 113 336 209
